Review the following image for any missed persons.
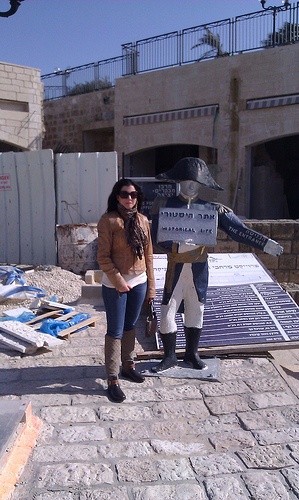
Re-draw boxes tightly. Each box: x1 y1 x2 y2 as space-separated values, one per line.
97 179 156 401
151 157 284 373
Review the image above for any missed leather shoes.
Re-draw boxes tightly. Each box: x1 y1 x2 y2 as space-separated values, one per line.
121 364 145 383
107 380 126 402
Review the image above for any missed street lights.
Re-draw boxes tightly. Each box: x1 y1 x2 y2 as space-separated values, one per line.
258 0 290 48
53 65 71 97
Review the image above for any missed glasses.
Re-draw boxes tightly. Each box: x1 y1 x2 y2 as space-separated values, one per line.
117 191 140 199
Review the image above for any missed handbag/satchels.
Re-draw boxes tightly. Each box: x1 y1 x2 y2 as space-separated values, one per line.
146 299 158 338
